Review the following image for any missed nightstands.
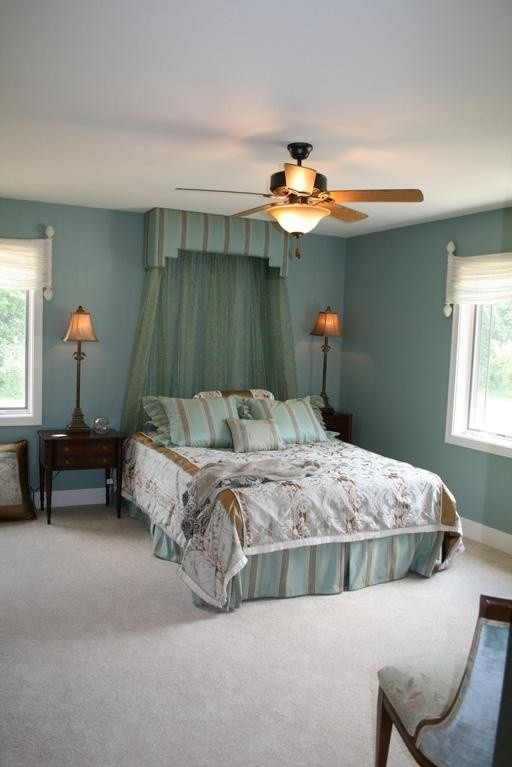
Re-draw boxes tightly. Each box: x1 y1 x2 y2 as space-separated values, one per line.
35 425 128 524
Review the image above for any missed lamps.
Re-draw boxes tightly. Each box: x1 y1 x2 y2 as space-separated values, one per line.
58 302 100 432
263 203 332 258
307 303 342 417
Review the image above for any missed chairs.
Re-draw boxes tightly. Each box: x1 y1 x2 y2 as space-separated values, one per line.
373 592 511 764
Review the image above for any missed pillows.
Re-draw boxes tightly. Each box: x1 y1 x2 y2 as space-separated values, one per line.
137 388 331 454
1 438 38 521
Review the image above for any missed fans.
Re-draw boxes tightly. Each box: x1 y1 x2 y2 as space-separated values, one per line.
173 142 424 225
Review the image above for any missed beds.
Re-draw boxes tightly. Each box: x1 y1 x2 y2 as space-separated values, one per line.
123 427 464 614
